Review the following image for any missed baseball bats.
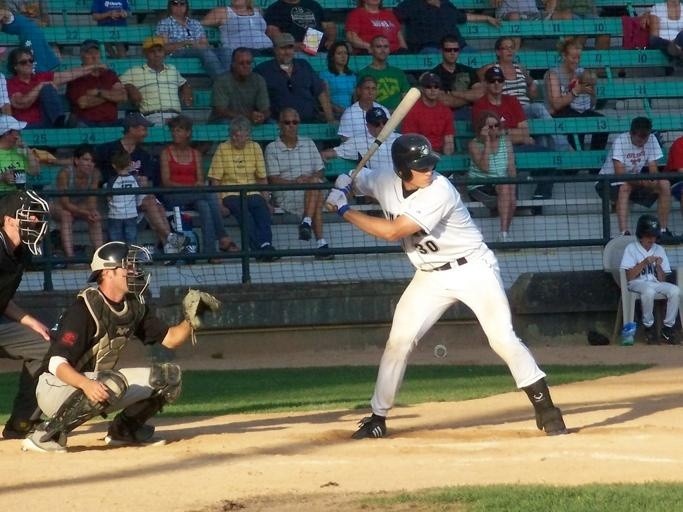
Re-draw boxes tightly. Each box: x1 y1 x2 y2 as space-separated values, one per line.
323 88 421 212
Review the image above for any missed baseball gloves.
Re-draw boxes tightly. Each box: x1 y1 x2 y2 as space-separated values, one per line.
180 286 223 334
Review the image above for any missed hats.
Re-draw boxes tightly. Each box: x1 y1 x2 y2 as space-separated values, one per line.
631 116 654 135
275 33 296 48
366 108 388 121
123 112 155 128
485 66 505 78
0 114 28 136
80 38 99 53
421 73 442 87
142 37 165 50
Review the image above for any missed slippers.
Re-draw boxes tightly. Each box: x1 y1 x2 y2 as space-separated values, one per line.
208 257 225 263
219 242 241 253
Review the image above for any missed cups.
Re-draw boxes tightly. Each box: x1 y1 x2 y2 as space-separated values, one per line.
14 168 27 189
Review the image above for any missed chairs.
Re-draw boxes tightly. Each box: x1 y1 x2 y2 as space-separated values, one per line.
602 235 682 344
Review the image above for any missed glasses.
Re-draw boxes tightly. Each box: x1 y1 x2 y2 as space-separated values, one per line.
170 0 186 5
282 119 298 125
488 123 500 130
486 78 505 84
371 121 387 127
17 57 34 65
443 47 460 53
424 84 441 90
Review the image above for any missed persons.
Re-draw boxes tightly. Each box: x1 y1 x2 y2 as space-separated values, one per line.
0 189 56 441
618 214 683 345
640 1 683 66
18 239 225 453
1 1 611 266
593 115 683 247
663 135 683 222
321 132 567 440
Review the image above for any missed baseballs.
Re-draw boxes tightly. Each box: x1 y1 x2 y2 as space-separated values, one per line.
433 345 447 360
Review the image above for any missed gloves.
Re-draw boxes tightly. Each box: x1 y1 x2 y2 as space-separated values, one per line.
324 189 349 216
335 173 355 194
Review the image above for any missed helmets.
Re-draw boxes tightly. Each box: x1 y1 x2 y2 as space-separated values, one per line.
636 214 663 238
391 134 442 182
87 240 154 305
2 190 49 257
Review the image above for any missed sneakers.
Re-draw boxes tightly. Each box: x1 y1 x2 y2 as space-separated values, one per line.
299 221 312 241
542 421 569 436
500 238 520 252
529 197 545 213
349 416 388 438
659 331 681 344
314 244 335 261
496 233 507 243
163 231 197 266
1 420 33 438
22 431 68 453
104 436 166 445
260 243 281 262
646 334 662 345
254 249 263 262
658 226 675 243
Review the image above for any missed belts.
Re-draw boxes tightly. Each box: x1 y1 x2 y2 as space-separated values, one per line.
431 258 466 270
143 110 182 115
